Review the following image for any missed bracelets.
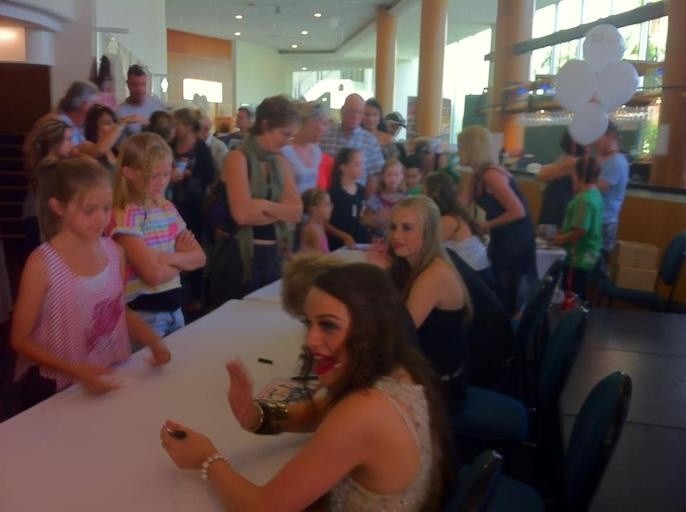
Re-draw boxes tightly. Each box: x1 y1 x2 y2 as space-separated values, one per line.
251 398 290 435
200 452 230 484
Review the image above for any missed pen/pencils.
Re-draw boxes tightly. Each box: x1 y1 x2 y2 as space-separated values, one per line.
168 428 185 439
291 376 318 380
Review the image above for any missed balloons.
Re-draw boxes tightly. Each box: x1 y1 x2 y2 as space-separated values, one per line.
599 62 638 108
584 26 627 79
555 58 597 112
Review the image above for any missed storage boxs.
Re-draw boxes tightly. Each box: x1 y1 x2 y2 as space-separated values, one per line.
613 264 659 293
609 240 659 270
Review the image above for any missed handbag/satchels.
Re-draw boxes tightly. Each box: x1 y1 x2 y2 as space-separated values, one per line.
201 181 238 234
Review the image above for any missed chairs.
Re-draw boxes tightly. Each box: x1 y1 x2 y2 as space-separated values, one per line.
454 305 587 456
438 370 635 511
458 254 567 388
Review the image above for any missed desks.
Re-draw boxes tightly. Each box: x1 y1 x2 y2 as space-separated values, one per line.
247 239 408 311
0 297 299 510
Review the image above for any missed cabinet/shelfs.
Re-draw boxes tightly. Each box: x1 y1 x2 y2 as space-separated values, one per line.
478 0 686 193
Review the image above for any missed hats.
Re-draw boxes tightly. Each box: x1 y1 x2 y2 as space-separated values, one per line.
386 112 407 129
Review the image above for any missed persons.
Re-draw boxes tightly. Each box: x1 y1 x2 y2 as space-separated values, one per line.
8 155 171 393
533 120 630 296
157 262 449 512
21 63 535 358
382 192 486 417
